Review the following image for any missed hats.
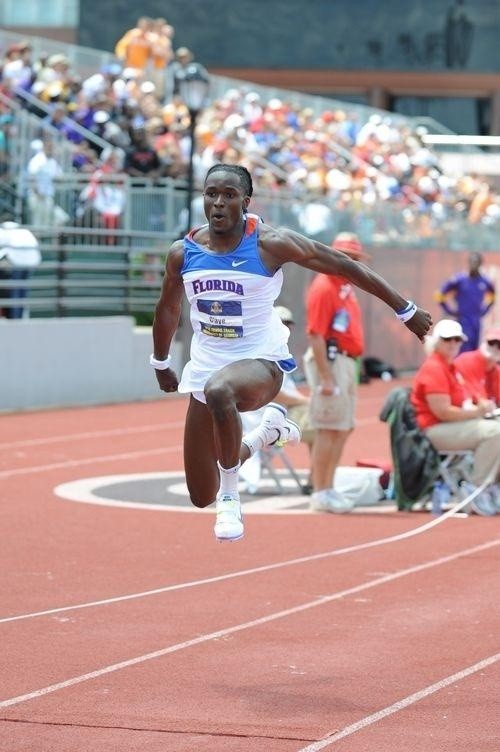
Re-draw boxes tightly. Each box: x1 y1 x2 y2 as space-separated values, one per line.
431 319 469 342
332 230 373 264
484 326 500 341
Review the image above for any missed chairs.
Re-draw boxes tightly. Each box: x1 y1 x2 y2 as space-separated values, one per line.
238 445 303 494
394 389 478 516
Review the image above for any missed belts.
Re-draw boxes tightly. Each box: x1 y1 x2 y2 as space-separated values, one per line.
336 350 359 362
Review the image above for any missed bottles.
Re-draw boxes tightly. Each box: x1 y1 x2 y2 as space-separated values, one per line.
431 481 442 517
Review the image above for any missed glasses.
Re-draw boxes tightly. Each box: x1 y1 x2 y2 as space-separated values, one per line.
445 338 462 342
488 340 500 349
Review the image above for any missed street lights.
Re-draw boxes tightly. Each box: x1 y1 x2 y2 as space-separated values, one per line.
176 62 211 228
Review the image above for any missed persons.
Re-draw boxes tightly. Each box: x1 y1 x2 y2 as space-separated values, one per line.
291 229 373 515
0 209 43 318
436 250 499 356
116 16 175 83
452 328 500 418
146 162 435 545
0 41 499 249
234 306 324 499
408 319 500 517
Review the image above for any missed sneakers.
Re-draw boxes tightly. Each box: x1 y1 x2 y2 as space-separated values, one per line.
212 489 245 541
460 480 500 516
308 487 355 514
262 402 303 443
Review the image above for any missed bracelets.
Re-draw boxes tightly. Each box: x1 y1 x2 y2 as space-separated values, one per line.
394 300 418 324
150 353 171 371
